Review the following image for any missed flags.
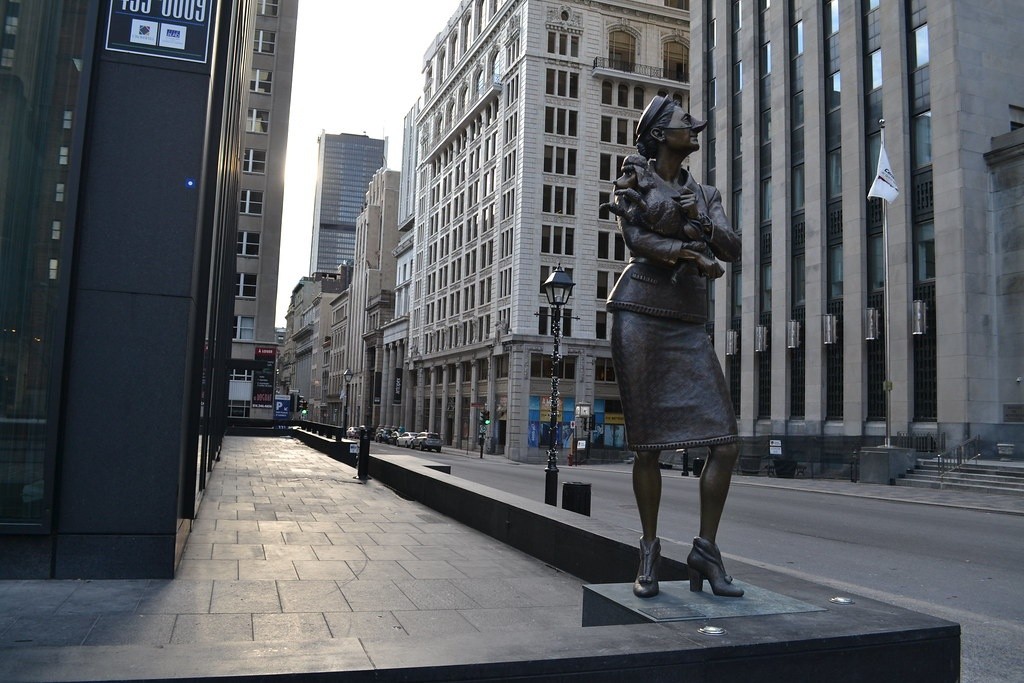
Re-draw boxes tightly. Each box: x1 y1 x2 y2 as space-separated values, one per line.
866 129 900 204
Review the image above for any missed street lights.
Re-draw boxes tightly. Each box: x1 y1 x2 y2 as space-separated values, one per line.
532 261 581 508
342 368 355 441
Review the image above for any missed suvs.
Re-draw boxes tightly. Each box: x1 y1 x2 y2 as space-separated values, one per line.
410 431 443 453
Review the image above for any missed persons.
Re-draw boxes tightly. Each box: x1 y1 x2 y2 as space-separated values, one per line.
605 94 746 598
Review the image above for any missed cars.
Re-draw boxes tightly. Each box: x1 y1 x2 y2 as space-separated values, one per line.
346 426 420 449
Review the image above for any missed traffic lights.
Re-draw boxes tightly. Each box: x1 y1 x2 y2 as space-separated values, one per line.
485 411 490 425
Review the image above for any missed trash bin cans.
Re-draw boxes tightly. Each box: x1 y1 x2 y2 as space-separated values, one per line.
562 480 592 517
692 458 705 477
